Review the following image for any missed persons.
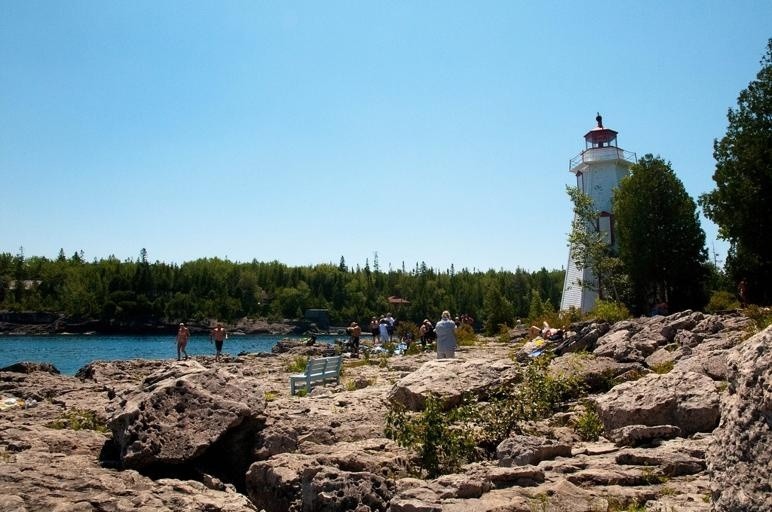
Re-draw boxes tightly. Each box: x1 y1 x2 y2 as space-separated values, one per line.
530 320 564 340
175 323 190 360
211 323 229 362
346 310 473 358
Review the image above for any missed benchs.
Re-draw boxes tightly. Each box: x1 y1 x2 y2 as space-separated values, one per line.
289 356 341 395
